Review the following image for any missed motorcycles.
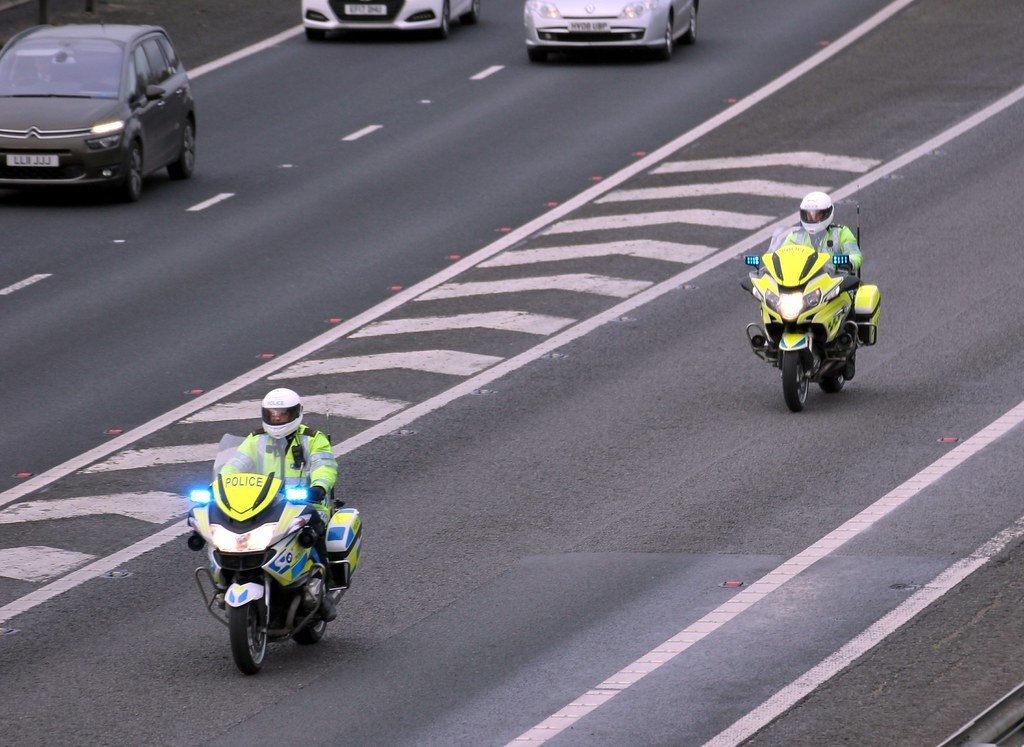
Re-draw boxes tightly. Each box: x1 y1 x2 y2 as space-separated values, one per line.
740 204 881 412
184 431 366 674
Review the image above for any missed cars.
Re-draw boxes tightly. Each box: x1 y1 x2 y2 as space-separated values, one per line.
298 1 480 41
522 1 699 63
0 21 199 204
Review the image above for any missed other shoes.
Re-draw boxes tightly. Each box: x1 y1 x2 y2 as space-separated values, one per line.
321 594 336 622
765 351 778 358
842 359 855 380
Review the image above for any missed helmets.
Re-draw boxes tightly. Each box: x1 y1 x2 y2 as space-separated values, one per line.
800 192 834 234
261 388 303 439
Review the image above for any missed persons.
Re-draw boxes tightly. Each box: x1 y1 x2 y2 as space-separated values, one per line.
758 192 864 380
185 388 338 622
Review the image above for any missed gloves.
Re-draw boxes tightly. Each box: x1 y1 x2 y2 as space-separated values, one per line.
838 263 853 271
308 486 326 501
